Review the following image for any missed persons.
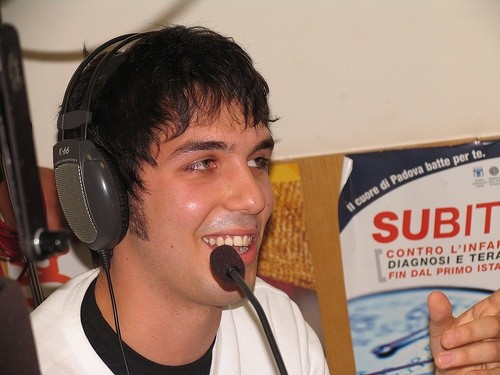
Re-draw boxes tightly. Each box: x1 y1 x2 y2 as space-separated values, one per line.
28 24 499 375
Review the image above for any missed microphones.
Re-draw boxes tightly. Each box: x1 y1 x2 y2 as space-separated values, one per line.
210 245 288 375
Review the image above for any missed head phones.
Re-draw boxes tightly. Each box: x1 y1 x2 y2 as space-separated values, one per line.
54 30 157 257
